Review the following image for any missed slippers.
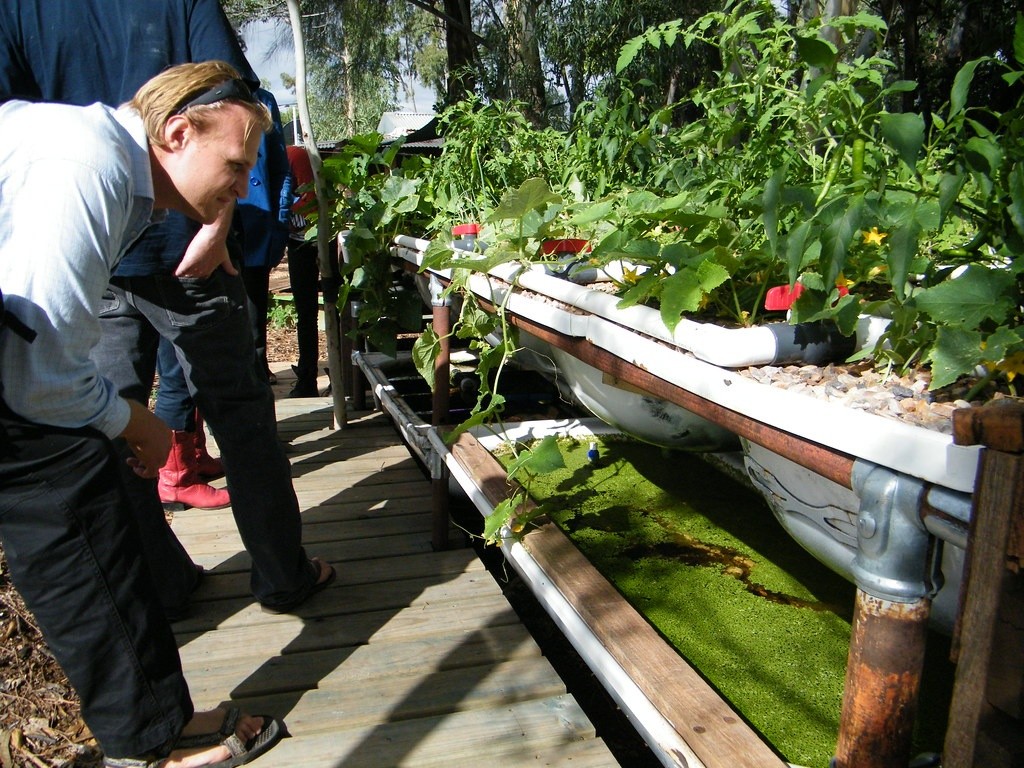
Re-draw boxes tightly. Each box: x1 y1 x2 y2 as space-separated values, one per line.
262 559 336 613
166 564 205 618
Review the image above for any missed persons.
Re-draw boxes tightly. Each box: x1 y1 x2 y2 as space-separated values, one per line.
0 59 278 768
286 146 346 398
237 88 294 385
0 0 338 614
153 334 231 510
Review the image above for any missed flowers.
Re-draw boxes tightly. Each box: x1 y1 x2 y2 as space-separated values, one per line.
448 168 862 325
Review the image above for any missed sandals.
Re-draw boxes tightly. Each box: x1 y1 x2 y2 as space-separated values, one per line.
103 701 286 768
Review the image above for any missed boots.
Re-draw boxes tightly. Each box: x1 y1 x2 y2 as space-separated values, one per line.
189 406 224 475
155 431 232 512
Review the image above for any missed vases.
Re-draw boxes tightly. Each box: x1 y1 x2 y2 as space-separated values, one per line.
394 233 843 368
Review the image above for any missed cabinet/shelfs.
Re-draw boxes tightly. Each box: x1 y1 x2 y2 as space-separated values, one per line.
339 226 1024 768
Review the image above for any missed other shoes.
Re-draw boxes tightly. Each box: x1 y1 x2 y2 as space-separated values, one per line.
268 368 276 384
